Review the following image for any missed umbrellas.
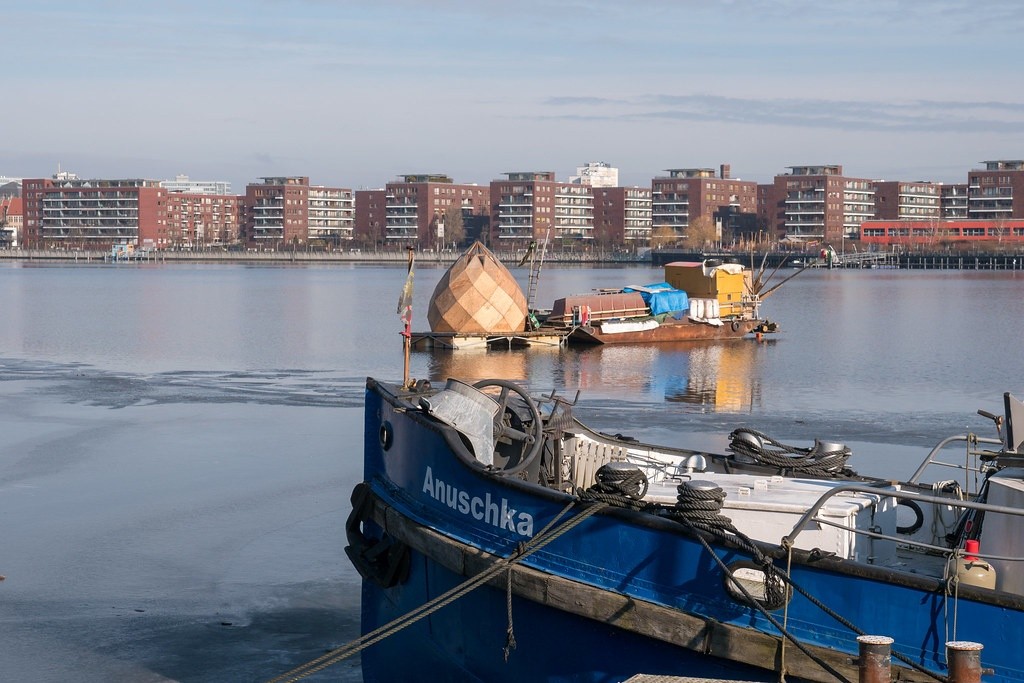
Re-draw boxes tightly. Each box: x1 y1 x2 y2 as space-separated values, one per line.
519 241 538 266
821 245 839 270
397 253 414 362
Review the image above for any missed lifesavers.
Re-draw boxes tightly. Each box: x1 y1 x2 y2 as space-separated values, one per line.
732 321 740 331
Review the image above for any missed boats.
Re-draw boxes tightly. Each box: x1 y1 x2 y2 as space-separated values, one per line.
554 259 779 345
354 368 1024 683
